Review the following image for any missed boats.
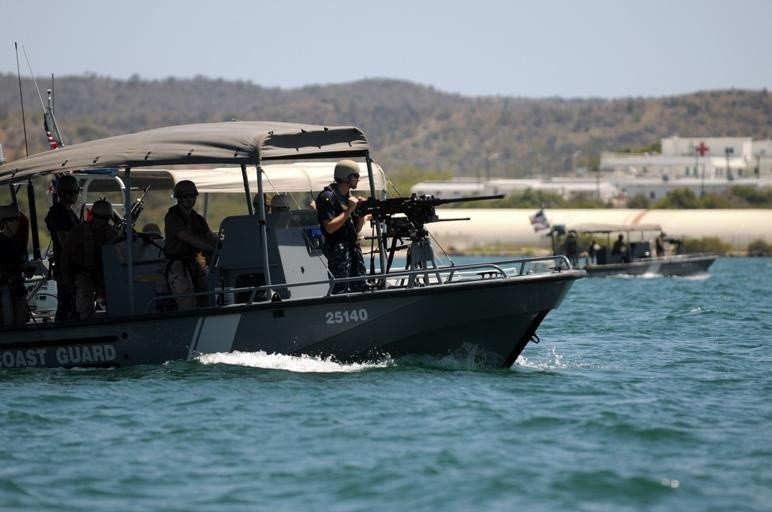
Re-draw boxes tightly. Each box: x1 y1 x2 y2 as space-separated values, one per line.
529 201 720 278
1 35 585 374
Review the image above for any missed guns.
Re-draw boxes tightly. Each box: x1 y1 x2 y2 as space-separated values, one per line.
355 194 503 237
97 184 152 232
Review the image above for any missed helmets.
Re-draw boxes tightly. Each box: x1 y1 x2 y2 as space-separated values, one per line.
55 174 79 190
0 205 21 219
270 195 291 209
252 193 271 206
92 200 114 217
334 160 360 179
143 223 161 233
173 180 199 196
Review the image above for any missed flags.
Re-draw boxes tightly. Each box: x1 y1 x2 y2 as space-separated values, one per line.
40 111 59 151
527 210 550 232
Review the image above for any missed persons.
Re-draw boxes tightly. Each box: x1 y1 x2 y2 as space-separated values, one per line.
0 203 37 328
588 240 597 264
162 179 223 310
314 158 374 297
613 234 629 262
252 193 271 214
140 222 164 241
44 175 83 322
655 232 667 257
563 230 579 265
267 193 292 215
63 199 119 321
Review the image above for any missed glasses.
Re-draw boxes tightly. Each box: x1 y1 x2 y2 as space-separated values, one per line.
4 216 22 223
180 192 196 200
